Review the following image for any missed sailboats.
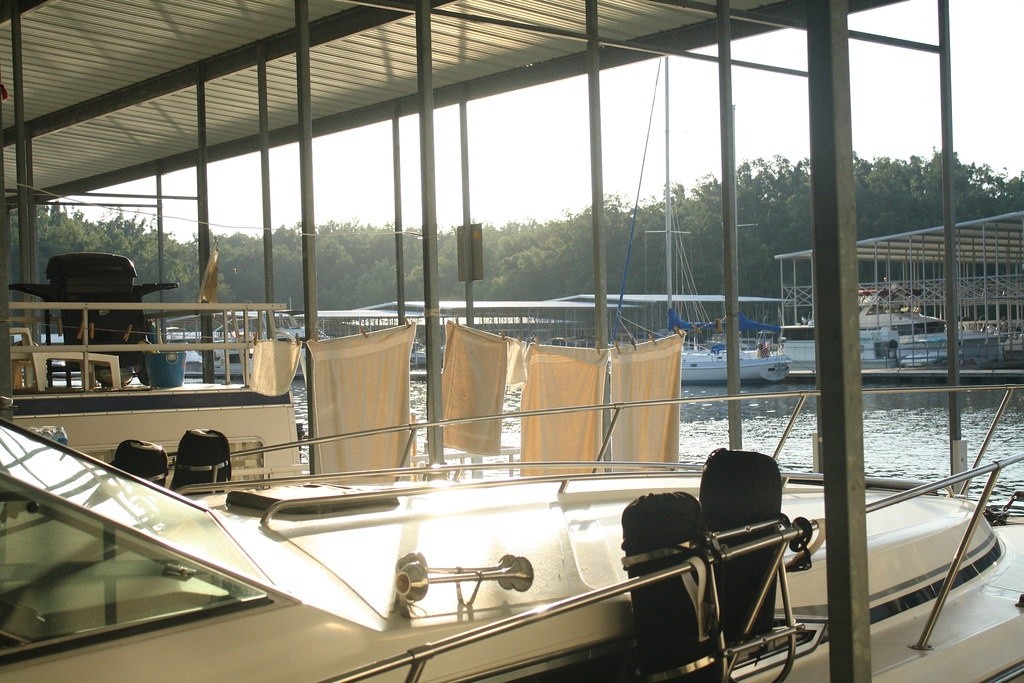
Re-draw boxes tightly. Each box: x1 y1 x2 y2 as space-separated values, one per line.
610 56 793 384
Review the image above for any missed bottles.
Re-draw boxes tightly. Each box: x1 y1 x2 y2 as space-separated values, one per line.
28 424 68 448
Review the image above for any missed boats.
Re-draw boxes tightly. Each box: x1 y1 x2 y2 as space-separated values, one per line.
1 383 1024 683
776 287 1008 369
15 311 331 381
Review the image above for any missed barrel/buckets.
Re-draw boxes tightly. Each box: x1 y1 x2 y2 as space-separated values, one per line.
145 351 187 388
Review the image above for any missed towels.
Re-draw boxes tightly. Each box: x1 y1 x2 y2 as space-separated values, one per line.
610 332 685 472
246 337 303 398
521 341 610 476
306 319 418 483
440 319 525 456
